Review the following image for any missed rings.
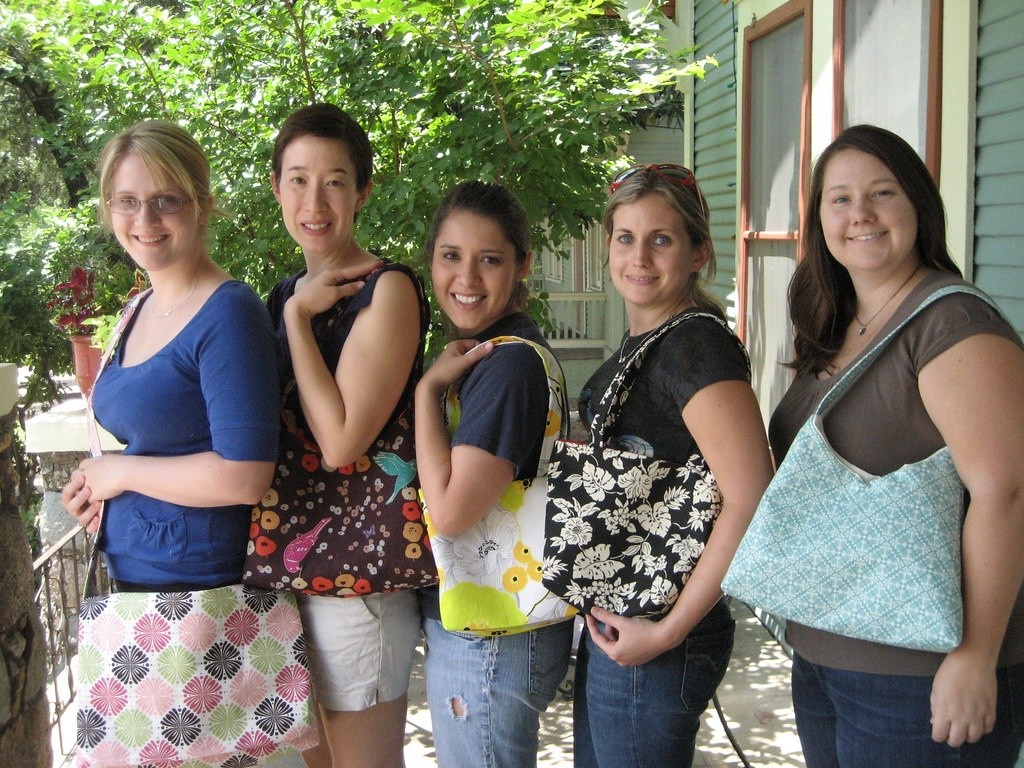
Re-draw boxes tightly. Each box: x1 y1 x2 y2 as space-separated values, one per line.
77 522 87 527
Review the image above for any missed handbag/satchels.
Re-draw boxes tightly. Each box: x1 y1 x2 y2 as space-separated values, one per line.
418 334 581 635
77 584 319 768
541 314 752 624
720 285 1024 654
245 261 439 598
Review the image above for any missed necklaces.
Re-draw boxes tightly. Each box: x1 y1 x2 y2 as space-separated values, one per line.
150 261 216 318
855 263 922 336
619 302 684 363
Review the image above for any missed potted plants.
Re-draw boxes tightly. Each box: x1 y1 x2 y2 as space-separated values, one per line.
48 265 151 400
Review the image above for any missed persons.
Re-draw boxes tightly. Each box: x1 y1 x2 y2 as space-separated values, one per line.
769 124 1023 768
414 180 577 768
62 117 320 768
572 162 774 768
268 101 432 768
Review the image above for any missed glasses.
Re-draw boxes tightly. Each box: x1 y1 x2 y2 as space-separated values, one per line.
106 195 201 214
610 165 705 220
